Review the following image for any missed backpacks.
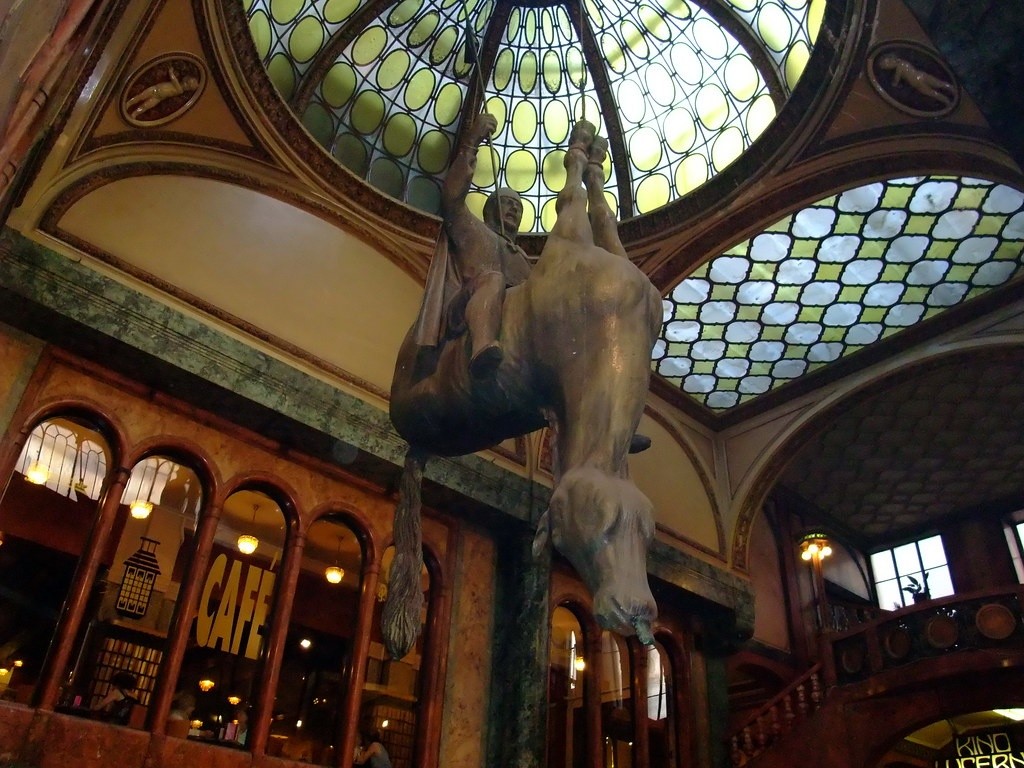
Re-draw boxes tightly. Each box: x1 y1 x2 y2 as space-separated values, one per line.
113 696 141 725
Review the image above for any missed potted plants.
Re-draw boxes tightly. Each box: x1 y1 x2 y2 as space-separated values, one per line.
901 572 931 603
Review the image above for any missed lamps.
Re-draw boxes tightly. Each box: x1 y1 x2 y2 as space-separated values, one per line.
800 546 811 560
227 696 242 705
821 542 832 556
238 504 259 555
325 535 345 584
199 680 215 691
129 500 154 520
807 540 818 553
24 462 51 485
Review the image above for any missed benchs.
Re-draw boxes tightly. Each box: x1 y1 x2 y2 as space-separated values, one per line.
167 718 190 738
126 705 147 730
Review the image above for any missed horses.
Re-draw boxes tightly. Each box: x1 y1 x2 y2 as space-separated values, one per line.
383 116 664 661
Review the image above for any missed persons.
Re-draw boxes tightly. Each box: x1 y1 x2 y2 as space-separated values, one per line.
280 723 391 767
858 608 871 622
94 672 138 726
170 677 251 749
440 116 654 453
0 633 21 702
